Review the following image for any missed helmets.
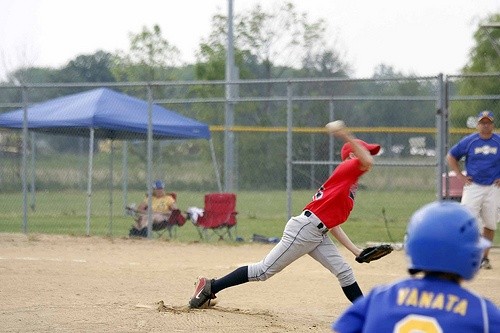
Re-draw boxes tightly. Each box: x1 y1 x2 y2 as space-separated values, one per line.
404 199 491 280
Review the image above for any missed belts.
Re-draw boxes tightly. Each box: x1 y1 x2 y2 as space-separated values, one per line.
304 210 329 235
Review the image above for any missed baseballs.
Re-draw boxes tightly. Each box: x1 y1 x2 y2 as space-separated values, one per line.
327 120 344 132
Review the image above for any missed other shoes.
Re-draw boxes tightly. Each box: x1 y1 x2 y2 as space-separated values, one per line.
189 276 216 310
481 257 491 271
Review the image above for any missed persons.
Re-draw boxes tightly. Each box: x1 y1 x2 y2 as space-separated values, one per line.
131 180 178 237
331 200 500 333
188 120 393 307
447 110 500 270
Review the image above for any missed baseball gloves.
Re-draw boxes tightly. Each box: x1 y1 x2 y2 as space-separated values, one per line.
355 245 393 264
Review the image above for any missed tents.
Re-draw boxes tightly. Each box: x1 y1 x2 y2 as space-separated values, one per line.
0 87 223 237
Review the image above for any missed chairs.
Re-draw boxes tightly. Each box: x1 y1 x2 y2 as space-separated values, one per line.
144 191 186 240
190 191 240 241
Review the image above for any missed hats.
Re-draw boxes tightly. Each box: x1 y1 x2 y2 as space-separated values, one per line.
153 180 165 190
341 139 381 160
477 111 496 123
